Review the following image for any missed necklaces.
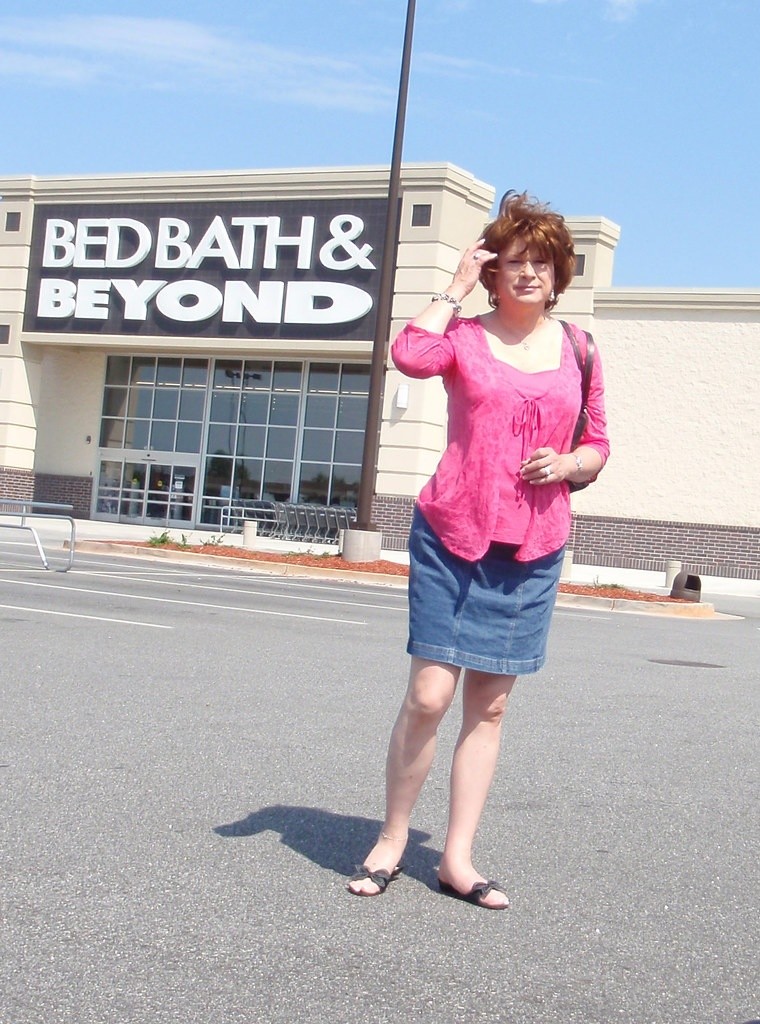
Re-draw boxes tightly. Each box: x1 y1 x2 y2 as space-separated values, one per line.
496 309 540 352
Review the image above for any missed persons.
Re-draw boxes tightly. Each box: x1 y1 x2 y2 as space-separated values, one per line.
346 189 610 911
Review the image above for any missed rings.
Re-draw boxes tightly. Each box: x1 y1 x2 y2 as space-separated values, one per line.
474 251 480 259
544 467 550 476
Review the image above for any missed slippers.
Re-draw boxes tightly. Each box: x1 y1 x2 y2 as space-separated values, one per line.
438 878 509 910
350 861 403 896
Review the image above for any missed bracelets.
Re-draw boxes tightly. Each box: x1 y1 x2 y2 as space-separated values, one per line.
432 291 462 318
569 452 583 477
